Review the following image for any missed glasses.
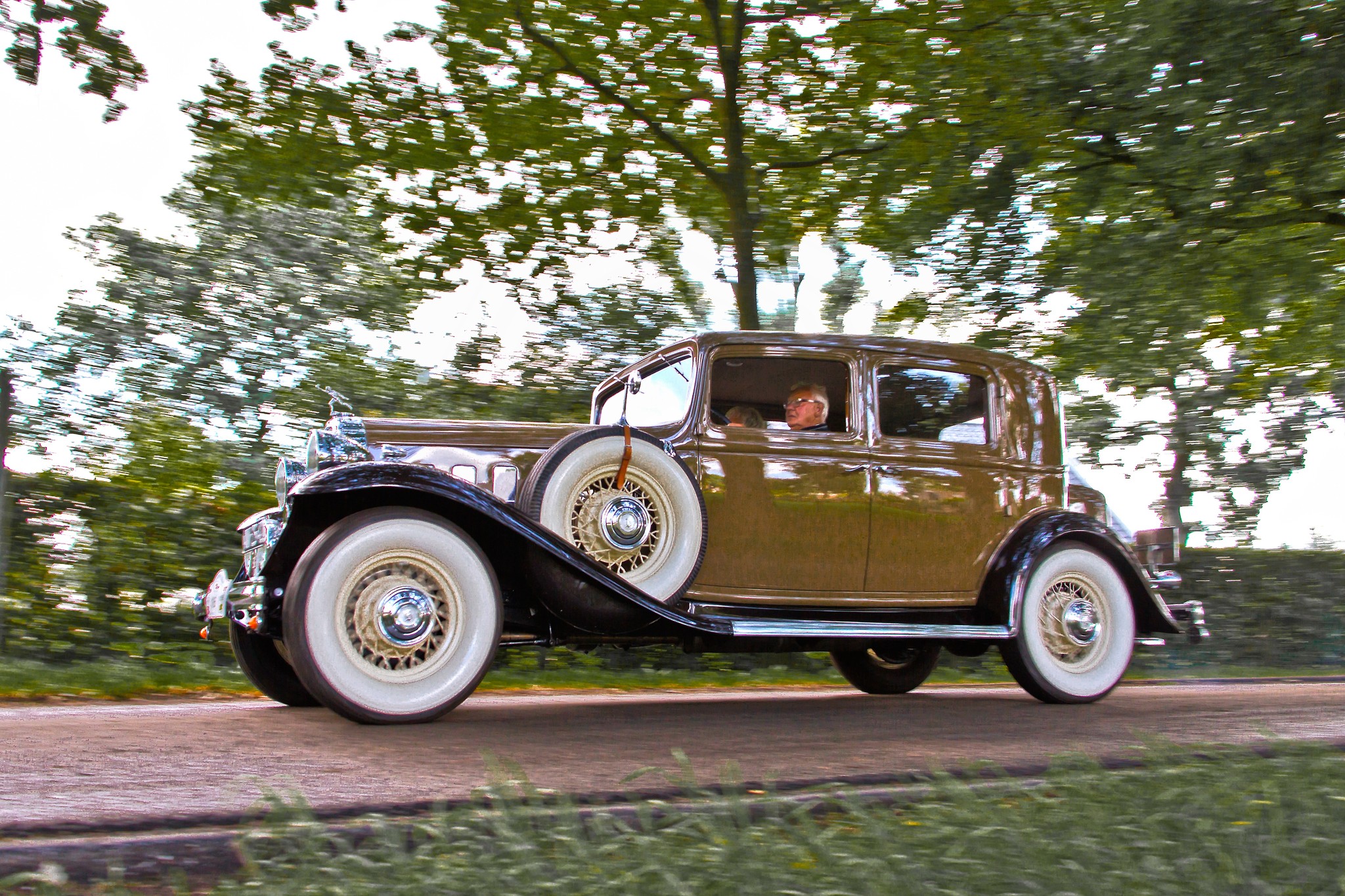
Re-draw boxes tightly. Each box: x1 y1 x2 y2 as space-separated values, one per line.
783 398 821 410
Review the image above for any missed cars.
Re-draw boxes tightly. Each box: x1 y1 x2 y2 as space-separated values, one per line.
203 330 1211 727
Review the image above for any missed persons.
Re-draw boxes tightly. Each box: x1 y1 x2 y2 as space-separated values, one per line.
722 382 831 432
316 386 353 416
